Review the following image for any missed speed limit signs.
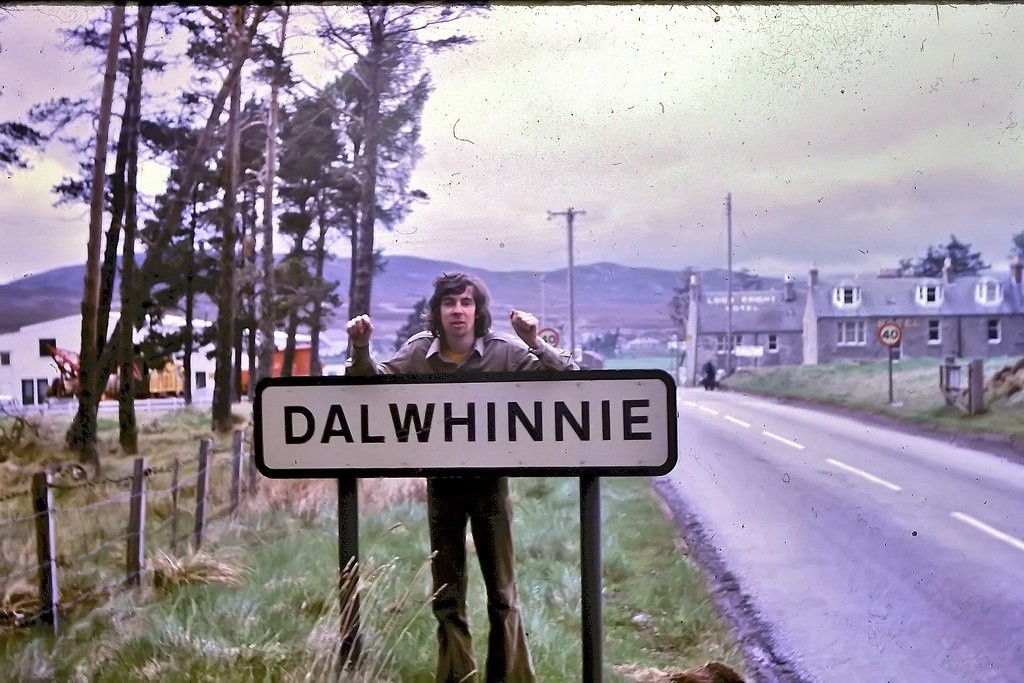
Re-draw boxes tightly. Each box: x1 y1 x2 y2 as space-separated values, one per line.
880 323 902 347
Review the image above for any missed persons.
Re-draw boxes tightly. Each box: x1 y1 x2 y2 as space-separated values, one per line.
700 356 716 391
342 270 581 683
